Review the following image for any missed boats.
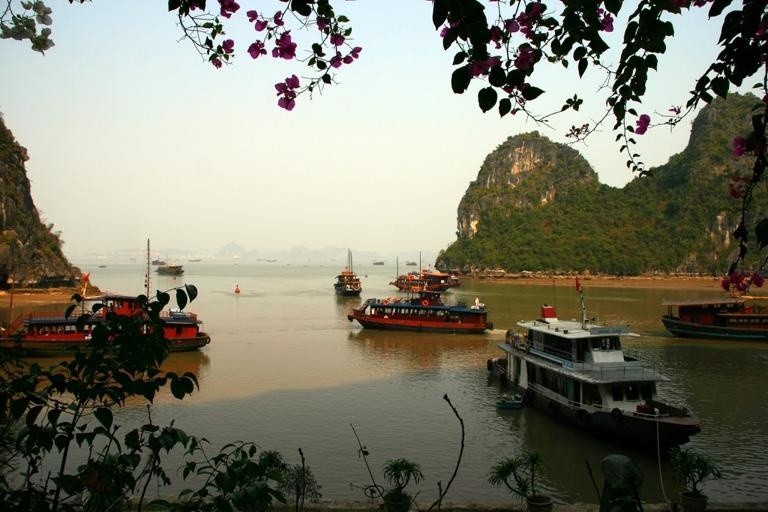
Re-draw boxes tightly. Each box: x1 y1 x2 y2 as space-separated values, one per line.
333 267 493 334
660 296 768 342
0 309 211 358
98 265 107 269
405 261 417 266
152 260 184 275
487 280 701 447
188 258 201 263
373 261 384 266
